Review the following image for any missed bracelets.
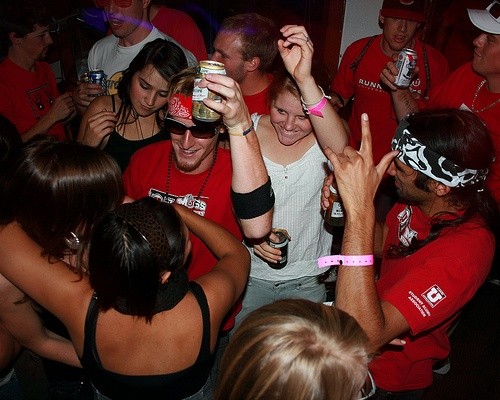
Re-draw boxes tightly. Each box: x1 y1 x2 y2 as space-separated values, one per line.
318 255 374 269
301 85 331 105
302 97 326 117
228 120 254 136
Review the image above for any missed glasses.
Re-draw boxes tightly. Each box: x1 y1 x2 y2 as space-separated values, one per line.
358 371 376 400
163 110 221 140
94 0 133 8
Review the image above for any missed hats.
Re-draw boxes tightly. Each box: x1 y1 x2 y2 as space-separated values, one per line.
380 0 426 23
467 0 500 35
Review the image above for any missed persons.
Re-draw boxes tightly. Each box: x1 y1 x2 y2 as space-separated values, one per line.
0 1 377 400
321 0 500 400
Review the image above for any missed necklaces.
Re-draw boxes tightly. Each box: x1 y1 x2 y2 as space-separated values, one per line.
166 139 218 211
471 80 500 112
135 112 156 140
